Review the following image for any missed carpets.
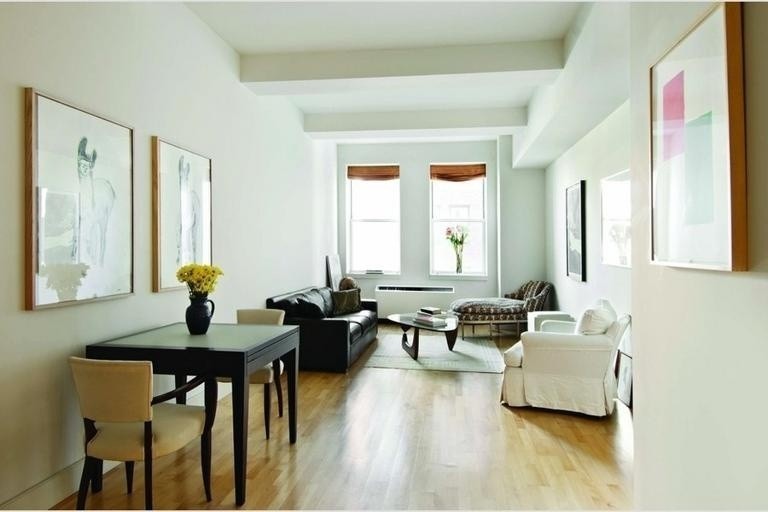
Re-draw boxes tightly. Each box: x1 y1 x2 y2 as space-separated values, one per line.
360 334 504 373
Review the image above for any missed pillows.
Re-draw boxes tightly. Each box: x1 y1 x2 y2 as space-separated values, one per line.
331 289 359 316
575 299 616 333
297 297 325 317
515 280 545 298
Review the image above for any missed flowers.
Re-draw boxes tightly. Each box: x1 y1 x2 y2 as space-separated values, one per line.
176 265 223 293
446 224 469 271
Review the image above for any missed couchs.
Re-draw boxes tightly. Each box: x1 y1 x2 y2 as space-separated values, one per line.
265 286 377 373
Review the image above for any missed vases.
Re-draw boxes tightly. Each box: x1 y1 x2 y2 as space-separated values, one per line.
186 293 215 335
454 245 462 273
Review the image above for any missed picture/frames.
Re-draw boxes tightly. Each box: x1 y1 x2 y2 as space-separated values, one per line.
23 88 134 307
566 180 587 282
617 352 633 408
152 137 212 293
647 2 750 272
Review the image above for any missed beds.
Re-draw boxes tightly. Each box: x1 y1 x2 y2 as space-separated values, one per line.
450 280 552 340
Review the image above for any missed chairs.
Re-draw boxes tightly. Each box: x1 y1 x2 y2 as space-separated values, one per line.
216 309 285 439
68 356 206 509
